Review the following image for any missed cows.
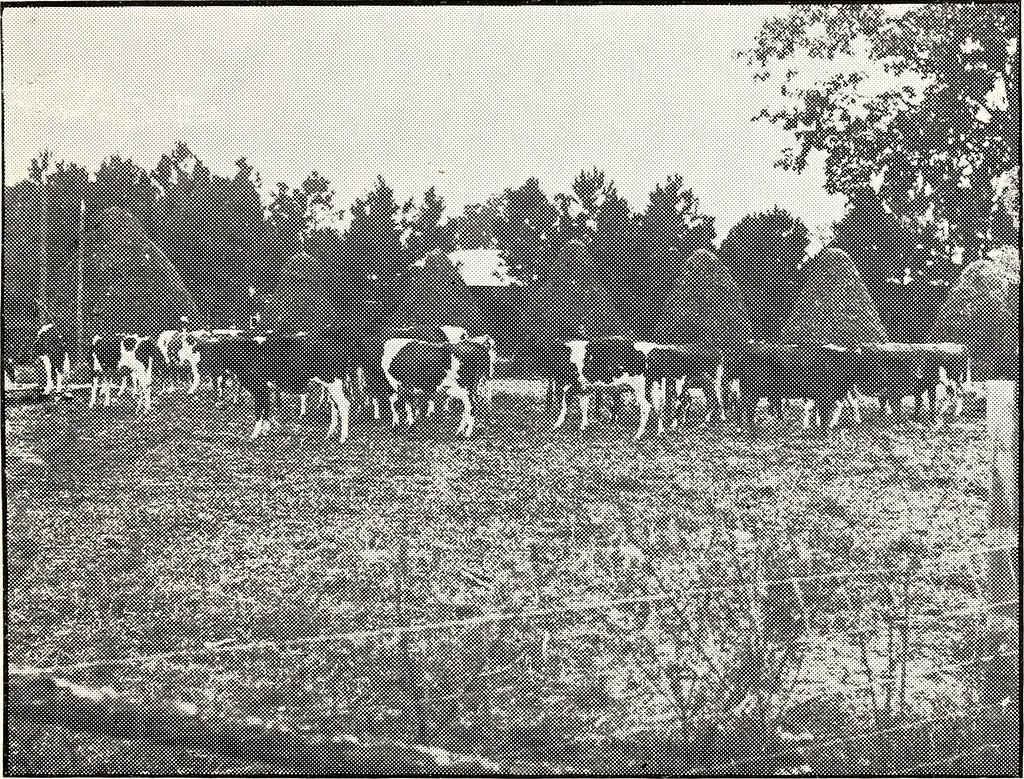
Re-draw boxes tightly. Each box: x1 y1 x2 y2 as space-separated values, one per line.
3 321 974 439
